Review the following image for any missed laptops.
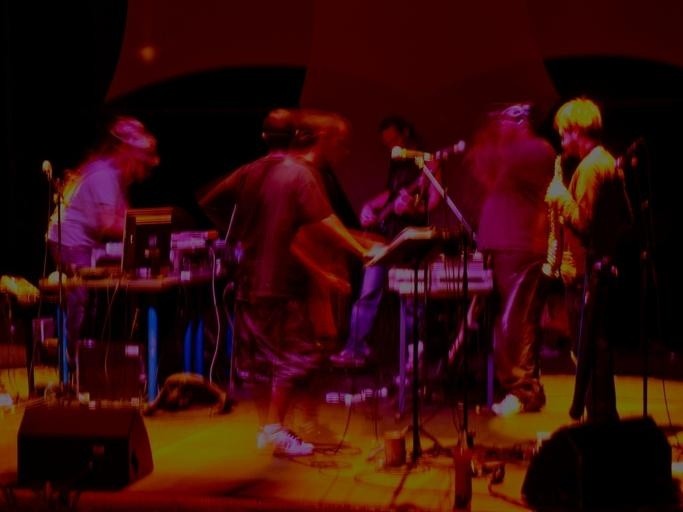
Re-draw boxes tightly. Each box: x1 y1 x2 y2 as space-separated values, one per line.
216 204 237 246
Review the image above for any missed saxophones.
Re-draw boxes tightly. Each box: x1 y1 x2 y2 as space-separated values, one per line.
542 152 564 278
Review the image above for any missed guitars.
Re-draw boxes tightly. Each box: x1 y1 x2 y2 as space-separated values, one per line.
366 141 467 236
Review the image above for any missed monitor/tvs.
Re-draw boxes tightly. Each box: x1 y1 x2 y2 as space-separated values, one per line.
119 207 176 280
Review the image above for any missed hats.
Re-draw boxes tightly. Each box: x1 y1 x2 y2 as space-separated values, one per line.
109 117 160 167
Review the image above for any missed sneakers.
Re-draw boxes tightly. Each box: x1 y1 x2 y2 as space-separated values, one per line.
330 350 366 368
258 422 314 457
492 394 522 415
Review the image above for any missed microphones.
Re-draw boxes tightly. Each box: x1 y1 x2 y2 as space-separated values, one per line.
42 160 53 179
391 146 433 161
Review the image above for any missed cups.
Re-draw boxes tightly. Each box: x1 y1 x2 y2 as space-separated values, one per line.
382 430 406 466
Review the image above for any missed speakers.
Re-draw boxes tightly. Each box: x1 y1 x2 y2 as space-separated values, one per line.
75 340 147 402
17 406 154 492
520 416 673 512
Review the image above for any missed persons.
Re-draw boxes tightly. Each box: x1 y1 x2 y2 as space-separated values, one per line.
45 115 160 396
461 101 557 418
326 112 443 388
542 94 646 423
195 109 386 456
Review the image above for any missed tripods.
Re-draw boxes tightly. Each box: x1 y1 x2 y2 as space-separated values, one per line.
367 164 523 511
